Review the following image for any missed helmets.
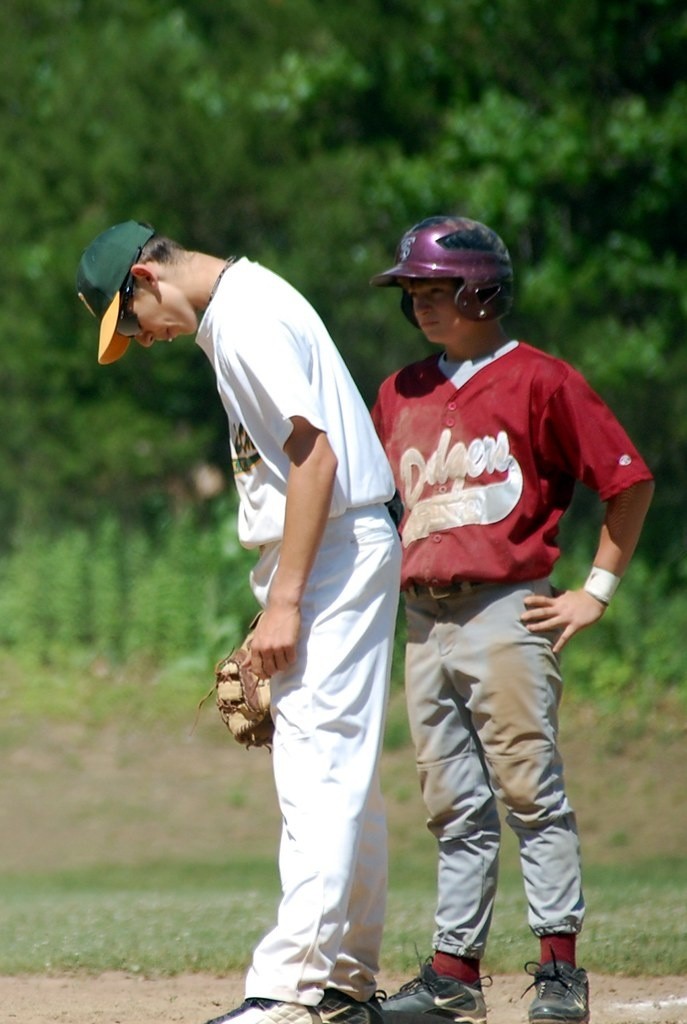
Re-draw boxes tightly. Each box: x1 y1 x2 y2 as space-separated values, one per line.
370 216 515 321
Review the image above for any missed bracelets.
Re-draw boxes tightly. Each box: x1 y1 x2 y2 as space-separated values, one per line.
584 567 620 606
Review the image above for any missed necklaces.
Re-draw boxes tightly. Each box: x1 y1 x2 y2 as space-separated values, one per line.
202 255 242 306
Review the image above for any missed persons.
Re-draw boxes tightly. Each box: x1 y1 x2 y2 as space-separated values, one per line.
76 217 402 1024
373 218 655 1024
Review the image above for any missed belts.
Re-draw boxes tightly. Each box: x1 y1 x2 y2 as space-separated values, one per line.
413 581 479 598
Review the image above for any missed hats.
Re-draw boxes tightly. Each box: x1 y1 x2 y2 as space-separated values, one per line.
76 219 155 365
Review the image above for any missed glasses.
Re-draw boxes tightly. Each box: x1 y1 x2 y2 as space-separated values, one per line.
116 247 144 339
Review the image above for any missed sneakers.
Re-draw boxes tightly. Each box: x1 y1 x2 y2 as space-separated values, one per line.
519 943 590 1024
204 996 322 1024
379 943 494 1024
314 987 386 1024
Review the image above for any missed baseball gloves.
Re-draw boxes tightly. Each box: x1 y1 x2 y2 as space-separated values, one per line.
213 630 275 748
